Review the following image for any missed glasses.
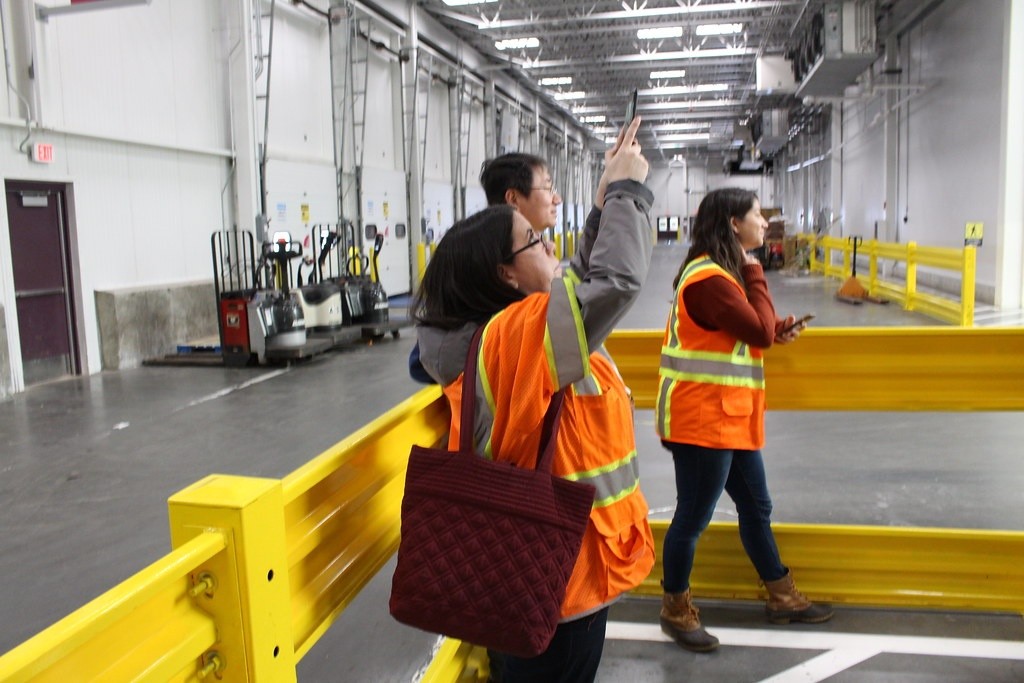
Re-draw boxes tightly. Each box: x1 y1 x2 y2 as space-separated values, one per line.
501 234 547 265
529 184 557 195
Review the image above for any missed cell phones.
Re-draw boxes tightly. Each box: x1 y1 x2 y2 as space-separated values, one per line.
623 88 638 139
778 313 816 338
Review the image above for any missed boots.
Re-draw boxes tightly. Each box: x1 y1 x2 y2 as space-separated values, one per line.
660 588 719 651
762 566 835 625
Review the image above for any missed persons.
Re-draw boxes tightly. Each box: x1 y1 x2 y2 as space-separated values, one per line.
413 115 657 683
653 187 836 652
408 152 564 385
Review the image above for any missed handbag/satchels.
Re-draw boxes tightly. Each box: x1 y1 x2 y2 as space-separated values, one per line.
388 320 599 658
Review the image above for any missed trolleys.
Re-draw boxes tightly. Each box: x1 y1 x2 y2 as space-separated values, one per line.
835 231 874 307
